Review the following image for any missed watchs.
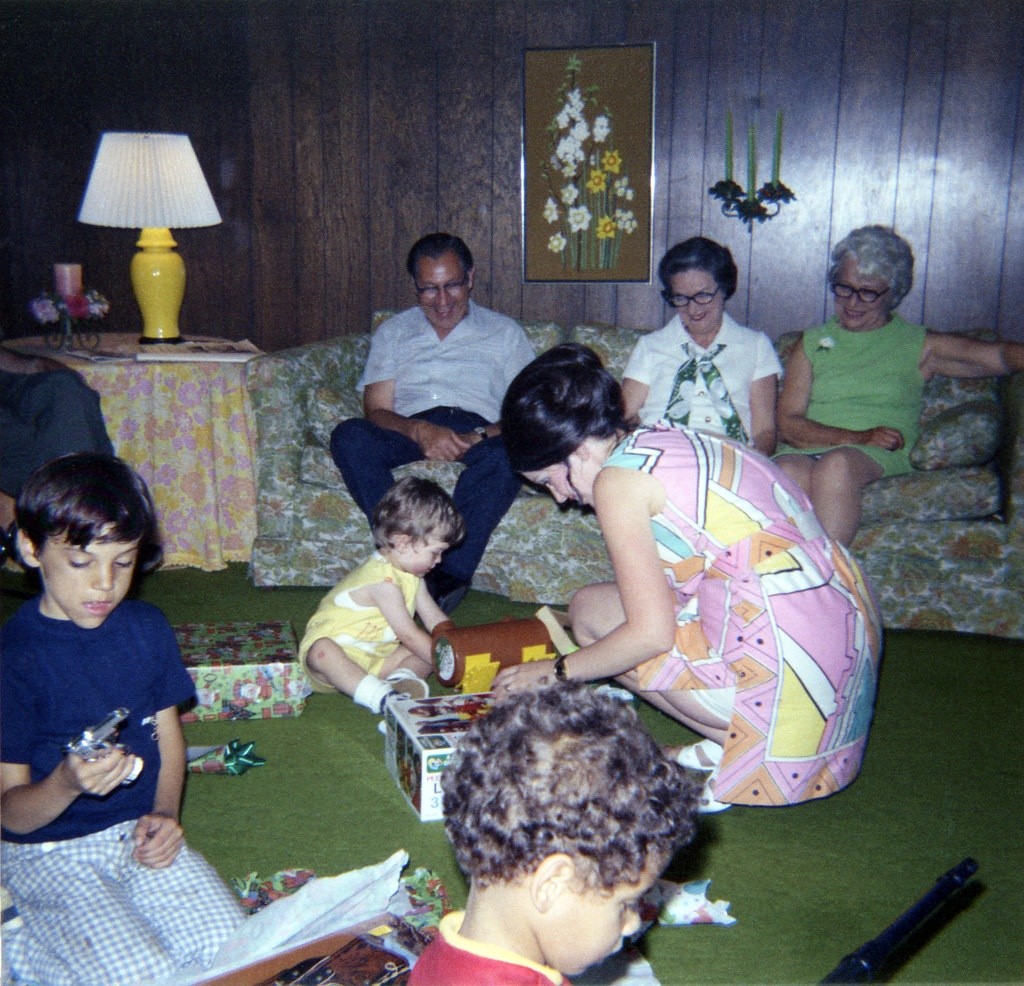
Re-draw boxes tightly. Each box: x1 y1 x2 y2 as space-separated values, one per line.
554 654 568 681
473 428 488 439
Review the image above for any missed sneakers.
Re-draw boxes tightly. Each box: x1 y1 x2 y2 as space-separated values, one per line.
372 676 430 720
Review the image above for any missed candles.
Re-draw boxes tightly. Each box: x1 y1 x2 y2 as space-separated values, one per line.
55 263 82 299
725 107 783 204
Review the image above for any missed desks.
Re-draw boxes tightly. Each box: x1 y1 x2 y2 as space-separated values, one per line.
0 333 263 570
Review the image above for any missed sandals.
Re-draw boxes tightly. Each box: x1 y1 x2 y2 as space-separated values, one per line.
660 736 734 812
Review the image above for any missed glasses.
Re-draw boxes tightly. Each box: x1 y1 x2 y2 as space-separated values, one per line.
831 283 890 303
413 273 467 297
667 284 721 307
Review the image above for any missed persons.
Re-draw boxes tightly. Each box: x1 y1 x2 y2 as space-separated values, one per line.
490 346 881 812
409 686 700 986
621 237 783 456
768 224 1024 550
2 347 245 986
297 475 466 734
329 232 539 614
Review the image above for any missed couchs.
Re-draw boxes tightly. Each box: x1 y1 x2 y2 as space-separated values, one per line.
242 310 1024 639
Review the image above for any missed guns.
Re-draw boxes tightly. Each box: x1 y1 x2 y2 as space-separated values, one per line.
62 705 143 784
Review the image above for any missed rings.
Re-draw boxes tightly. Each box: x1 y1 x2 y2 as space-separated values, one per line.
504 685 511 693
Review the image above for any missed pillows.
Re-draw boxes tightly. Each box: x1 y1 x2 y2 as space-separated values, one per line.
909 397 1004 470
306 364 365 450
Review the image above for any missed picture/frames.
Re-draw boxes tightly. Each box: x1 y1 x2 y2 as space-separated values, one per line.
520 41 656 285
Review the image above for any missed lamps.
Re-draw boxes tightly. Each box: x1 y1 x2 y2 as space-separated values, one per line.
77 131 223 345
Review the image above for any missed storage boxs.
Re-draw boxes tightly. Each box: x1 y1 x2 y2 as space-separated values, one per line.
170 622 306 722
385 691 497 822
